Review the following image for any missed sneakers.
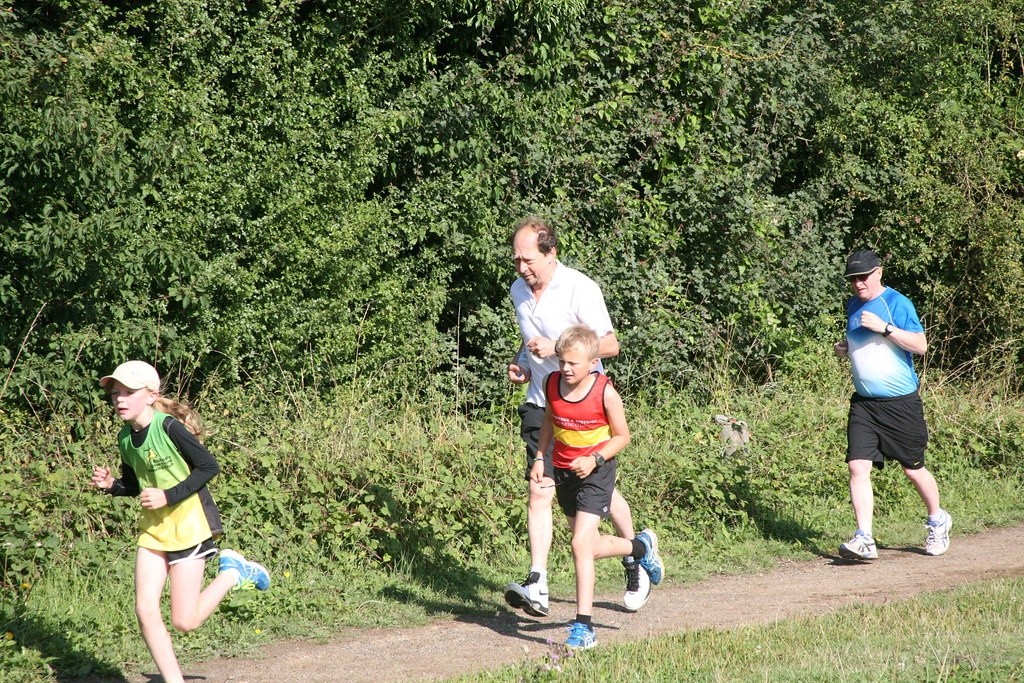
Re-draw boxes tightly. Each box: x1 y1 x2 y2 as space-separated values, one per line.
838 532 879 561
634 528 665 586
621 555 651 612
924 510 954 557
218 548 270 593
503 571 550 618
563 622 597 653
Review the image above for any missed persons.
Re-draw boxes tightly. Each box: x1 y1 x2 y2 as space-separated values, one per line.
834 249 953 560
505 216 652 617
88 360 269 683
528 324 665 653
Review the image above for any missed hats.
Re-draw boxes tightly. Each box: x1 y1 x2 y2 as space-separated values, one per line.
100 360 161 395
843 248 882 278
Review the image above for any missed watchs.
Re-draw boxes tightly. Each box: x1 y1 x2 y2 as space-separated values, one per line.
882 323 894 337
591 452 605 465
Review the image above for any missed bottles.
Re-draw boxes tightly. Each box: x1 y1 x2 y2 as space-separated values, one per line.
516 344 529 376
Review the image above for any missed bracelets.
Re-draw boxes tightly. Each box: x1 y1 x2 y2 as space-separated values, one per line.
555 340 559 356
533 458 545 461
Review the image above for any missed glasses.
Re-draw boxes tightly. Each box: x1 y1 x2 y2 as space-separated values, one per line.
845 269 876 282
